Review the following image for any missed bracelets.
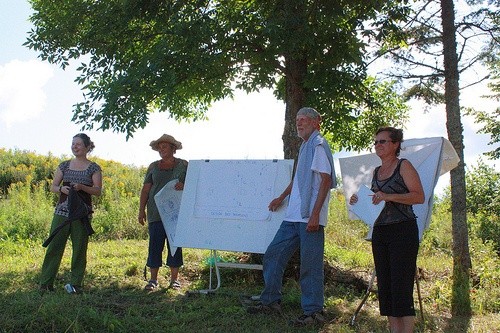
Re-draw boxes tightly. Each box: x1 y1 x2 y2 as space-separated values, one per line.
59 186 63 192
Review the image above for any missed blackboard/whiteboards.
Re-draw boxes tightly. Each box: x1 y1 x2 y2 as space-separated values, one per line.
172 159 295 254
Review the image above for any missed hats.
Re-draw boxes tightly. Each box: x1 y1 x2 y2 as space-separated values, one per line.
149 134 182 151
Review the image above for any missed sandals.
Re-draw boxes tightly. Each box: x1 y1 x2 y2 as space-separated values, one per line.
145 280 158 291
169 280 181 289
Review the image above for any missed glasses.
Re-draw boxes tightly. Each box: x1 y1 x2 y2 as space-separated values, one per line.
374 140 396 145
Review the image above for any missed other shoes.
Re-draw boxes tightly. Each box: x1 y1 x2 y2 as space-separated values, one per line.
247 302 282 313
73 284 83 294
289 313 323 327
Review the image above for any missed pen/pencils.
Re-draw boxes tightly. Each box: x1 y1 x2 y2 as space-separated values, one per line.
368 194 375 196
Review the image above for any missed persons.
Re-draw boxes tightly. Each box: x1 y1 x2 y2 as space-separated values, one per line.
38 133 103 295
138 134 189 291
349 127 425 333
248 108 337 326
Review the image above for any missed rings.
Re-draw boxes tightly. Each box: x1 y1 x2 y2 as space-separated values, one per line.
377 197 379 200
